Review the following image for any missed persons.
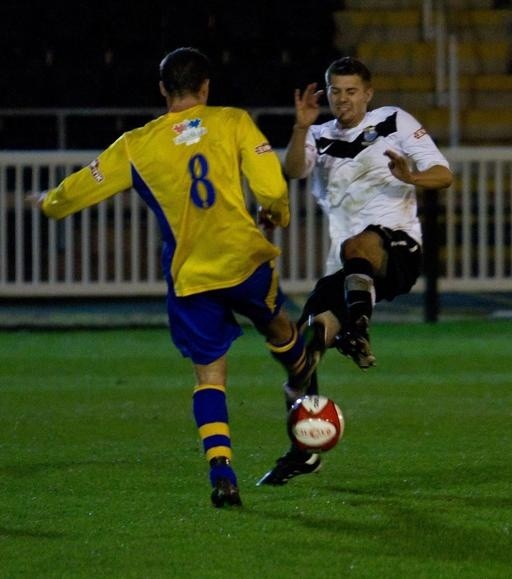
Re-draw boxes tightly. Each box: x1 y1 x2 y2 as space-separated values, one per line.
253 54 455 484
6 45 326 506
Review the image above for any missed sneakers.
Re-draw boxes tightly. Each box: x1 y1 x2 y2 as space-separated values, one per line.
256 449 322 487
210 477 243 507
288 321 327 391
335 330 376 375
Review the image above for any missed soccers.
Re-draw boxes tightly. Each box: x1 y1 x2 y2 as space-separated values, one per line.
287 396 344 451
